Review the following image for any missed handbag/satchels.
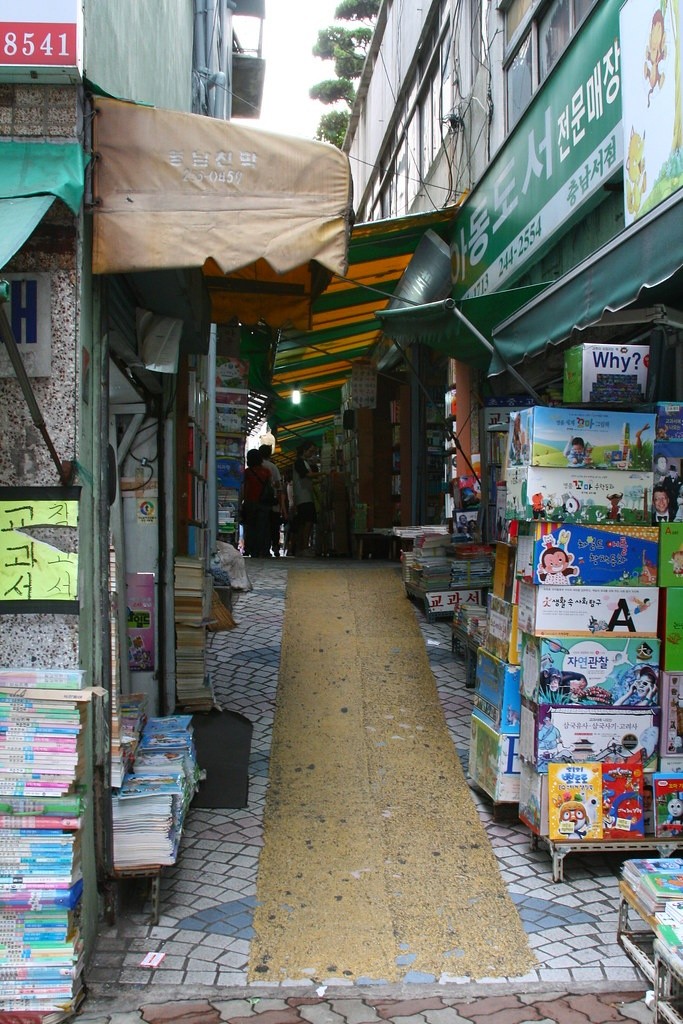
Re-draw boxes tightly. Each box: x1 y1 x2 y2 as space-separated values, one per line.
261 480 276 507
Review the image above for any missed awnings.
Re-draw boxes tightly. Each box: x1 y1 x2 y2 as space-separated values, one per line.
270 204 469 474
485 181 683 381
92 99 349 276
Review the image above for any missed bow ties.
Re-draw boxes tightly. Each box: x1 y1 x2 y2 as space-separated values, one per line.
657 516 667 522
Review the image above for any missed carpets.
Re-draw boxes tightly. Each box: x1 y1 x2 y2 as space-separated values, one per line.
174 709 254 808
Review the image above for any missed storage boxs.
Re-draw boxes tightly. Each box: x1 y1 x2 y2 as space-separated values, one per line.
469 348 683 837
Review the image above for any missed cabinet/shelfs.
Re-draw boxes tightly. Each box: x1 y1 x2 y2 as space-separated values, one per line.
315 473 350 557
389 400 452 561
342 408 373 530
173 350 210 559
483 428 509 547
215 387 251 550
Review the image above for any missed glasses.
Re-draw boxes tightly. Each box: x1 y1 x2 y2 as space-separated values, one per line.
637 679 652 688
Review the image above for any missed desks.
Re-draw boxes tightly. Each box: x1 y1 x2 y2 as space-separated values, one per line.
352 528 396 560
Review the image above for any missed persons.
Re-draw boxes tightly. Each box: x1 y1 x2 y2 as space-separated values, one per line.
650 487 676 522
562 435 593 465
243 442 320 558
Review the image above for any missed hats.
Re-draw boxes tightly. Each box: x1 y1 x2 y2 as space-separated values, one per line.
640 666 660 683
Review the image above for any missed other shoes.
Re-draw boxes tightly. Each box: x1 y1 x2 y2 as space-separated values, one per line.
296 551 315 558
